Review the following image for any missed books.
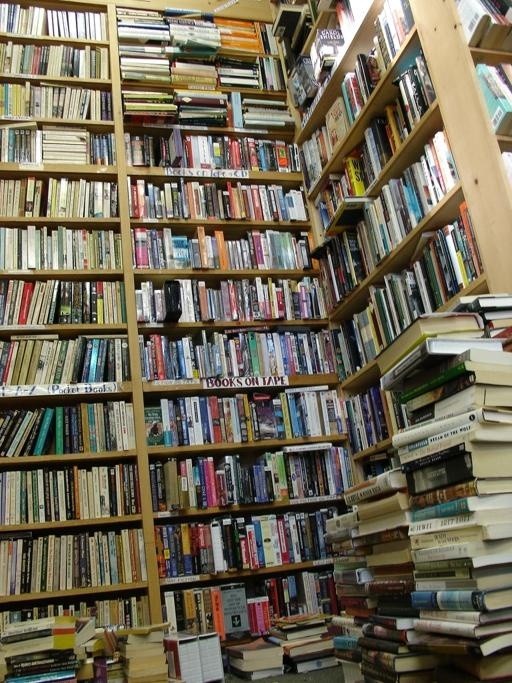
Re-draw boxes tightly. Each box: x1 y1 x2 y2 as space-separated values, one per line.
117 5 296 134
125 129 320 270
2 401 141 526
271 1 437 229
1 278 131 385
136 274 348 449
3 528 169 683
147 443 356 683
2 82 117 166
1 175 123 272
2 1 110 80
309 131 512 683
453 1 511 177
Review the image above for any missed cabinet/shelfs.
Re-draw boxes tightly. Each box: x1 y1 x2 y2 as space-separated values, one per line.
112 0 356 683
283 1 512 493
471 44 512 140
1 0 167 683
